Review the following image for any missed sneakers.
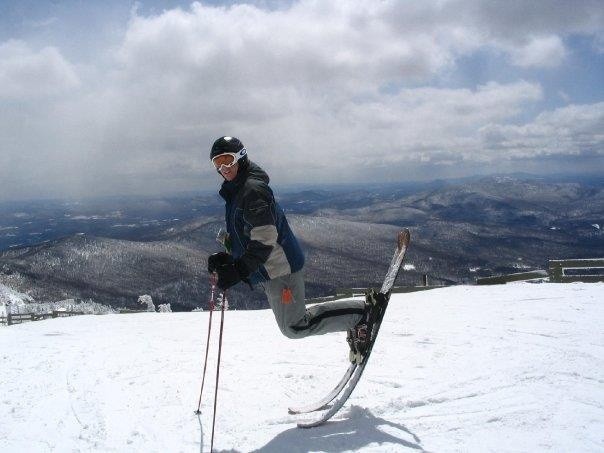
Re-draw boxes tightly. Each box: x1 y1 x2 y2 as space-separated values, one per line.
353 302 375 356
346 328 357 354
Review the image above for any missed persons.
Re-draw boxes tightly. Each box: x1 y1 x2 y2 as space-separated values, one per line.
208 137 387 365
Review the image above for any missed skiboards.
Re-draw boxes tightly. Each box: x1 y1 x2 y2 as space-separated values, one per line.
287 228 410 429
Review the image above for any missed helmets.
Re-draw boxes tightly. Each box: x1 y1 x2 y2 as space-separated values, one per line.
210 136 248 167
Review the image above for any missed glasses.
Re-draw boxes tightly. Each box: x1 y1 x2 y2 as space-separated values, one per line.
211 147 246 171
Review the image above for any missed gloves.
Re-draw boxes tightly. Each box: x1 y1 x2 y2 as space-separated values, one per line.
217 256 253 290
207 251 224 274
